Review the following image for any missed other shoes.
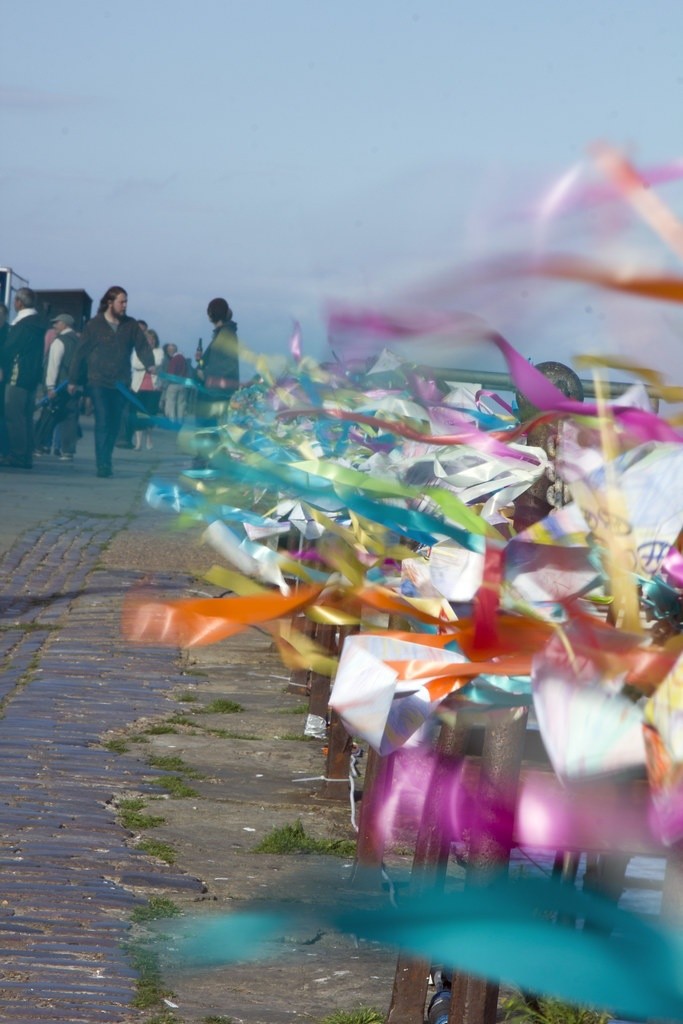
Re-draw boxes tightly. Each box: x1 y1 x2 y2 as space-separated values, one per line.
0 457 32 469
59 453 74 460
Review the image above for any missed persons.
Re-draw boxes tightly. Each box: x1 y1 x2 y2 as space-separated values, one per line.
192 297 240 469
67 286 157 478
0 287 187 468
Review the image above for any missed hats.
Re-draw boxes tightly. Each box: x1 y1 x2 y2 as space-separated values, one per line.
49 314 75 326
